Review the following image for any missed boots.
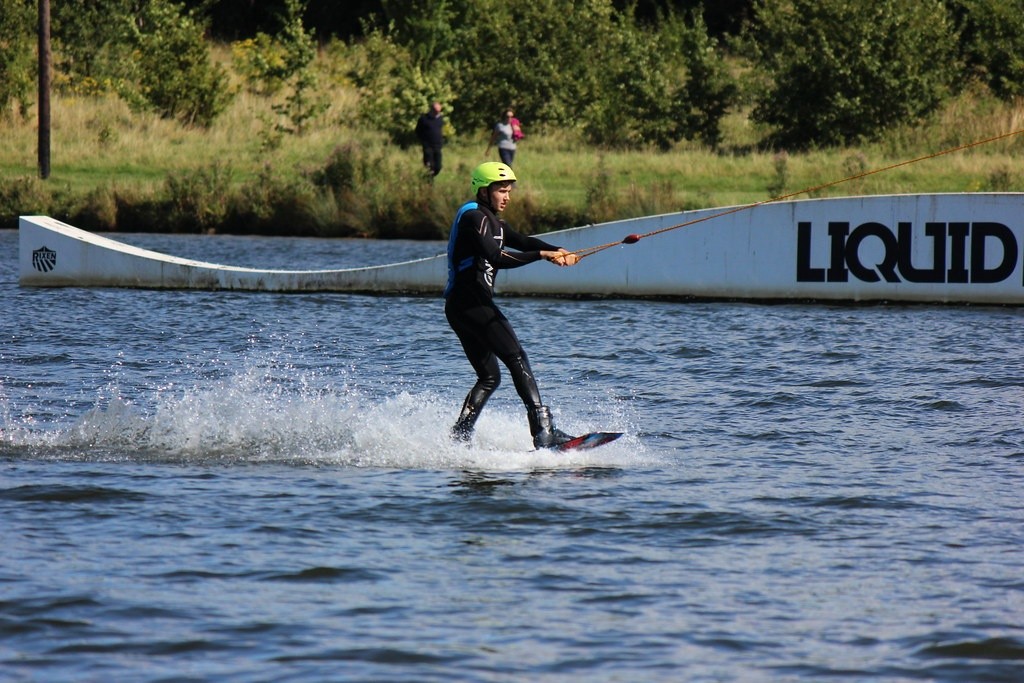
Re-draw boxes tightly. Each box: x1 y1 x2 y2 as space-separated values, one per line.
528 406 577 449
448 405 479 449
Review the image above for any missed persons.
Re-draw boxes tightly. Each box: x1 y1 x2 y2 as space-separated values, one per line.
485 108 518 169
414 94 444 178
444 162 578 450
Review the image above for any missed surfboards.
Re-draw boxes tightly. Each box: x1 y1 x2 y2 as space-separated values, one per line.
528 430 626 454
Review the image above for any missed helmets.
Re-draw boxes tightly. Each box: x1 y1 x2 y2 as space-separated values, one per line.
469 161 518 196
